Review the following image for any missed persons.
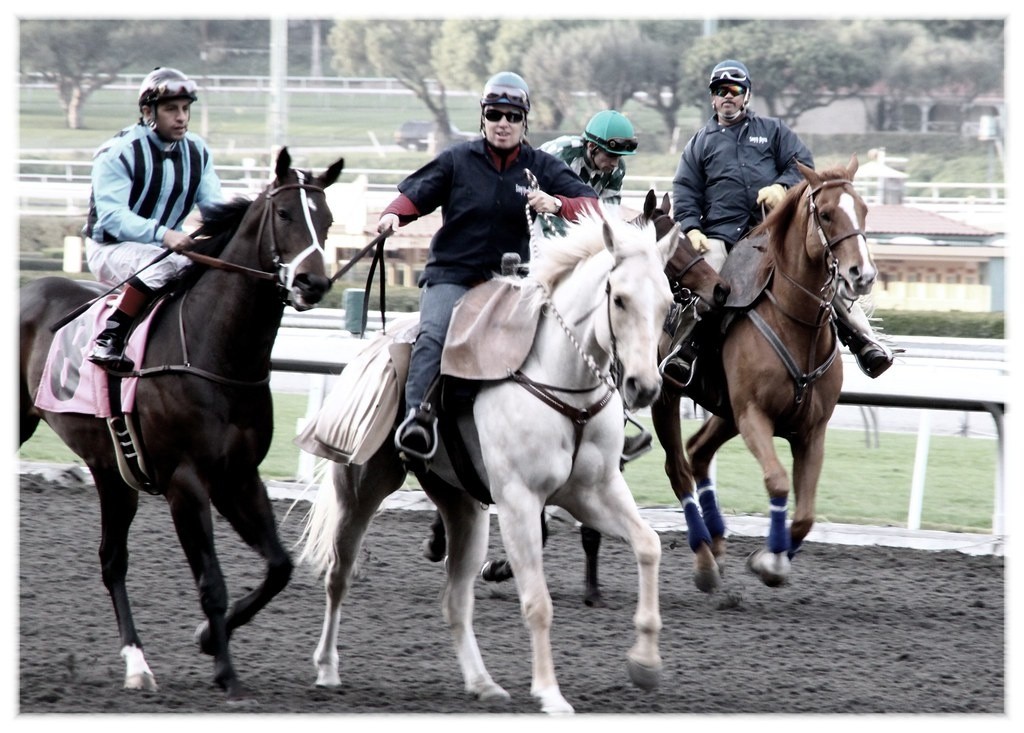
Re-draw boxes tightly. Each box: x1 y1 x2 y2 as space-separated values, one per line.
378 72 598 469
81 72 228 378
527 107 638 235
664 58 890 383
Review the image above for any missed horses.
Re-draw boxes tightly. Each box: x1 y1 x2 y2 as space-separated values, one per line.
417 189 732 611
655 151 892 594
19 146 345 711
280 189 680 715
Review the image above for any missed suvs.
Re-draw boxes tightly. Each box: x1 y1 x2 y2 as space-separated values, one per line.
395 121 479 153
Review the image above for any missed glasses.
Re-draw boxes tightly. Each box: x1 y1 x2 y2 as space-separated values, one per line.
713 86 746 97
482 85 530 110
482 109 524 123
710 67 747 81
606 137 638 153
158 79 198 95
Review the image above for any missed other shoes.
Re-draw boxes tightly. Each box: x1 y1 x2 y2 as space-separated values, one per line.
402 424 429 475
620 431 652 471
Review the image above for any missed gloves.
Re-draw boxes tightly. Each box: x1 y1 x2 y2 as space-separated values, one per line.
686 228 710 254
757 184 786 210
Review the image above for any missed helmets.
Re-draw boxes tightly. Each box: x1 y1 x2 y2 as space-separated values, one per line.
709 60 751 91
479 71 530 111
139 68 197 107
583 110 638 155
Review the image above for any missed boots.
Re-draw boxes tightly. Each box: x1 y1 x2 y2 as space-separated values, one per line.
831 294 887 373
87 275 156 373
665 292 712 396
